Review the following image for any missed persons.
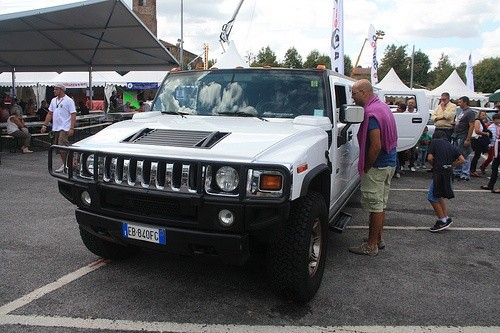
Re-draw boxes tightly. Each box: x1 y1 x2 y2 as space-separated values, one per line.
427 131 465 232
139 101 145 112
349 79 398 255
41 83 77 173
7 107 34 153
0 98 90 124
388 93 500 194
110 91 131 112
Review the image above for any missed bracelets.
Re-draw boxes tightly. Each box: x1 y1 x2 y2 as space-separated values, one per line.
70 128 75 130
42 125 47 129
466 139 470 141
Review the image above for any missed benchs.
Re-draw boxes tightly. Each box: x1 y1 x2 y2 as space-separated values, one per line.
1 133 50 153
74 122 112 142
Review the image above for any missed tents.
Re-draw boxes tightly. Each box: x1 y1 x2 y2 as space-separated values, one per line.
0 71 172 108
431 69 485 107
372 67 411 91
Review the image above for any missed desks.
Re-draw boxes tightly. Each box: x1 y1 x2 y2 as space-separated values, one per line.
108 112 134 120
76 114 105 136
0 122 51 148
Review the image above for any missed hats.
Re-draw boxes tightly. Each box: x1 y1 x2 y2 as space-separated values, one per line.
54 83 66 92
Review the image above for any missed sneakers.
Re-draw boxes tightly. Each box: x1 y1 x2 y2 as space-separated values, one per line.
429 217 453 232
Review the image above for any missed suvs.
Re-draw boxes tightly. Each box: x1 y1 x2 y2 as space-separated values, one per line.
47 65 432 306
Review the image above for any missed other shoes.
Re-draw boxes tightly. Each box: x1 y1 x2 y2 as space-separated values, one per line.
348 242 379 256
363 236 386 249
491 189 500 193
55 164 67 172
23 147 33 153
480 185 494 190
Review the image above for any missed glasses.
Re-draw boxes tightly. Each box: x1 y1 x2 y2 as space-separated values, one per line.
439 97 449 101
351 89 367 96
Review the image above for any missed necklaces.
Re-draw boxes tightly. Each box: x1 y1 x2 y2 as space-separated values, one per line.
56 98 63 108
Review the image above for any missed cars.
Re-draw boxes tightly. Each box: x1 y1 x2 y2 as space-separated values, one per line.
421 105 498 152
